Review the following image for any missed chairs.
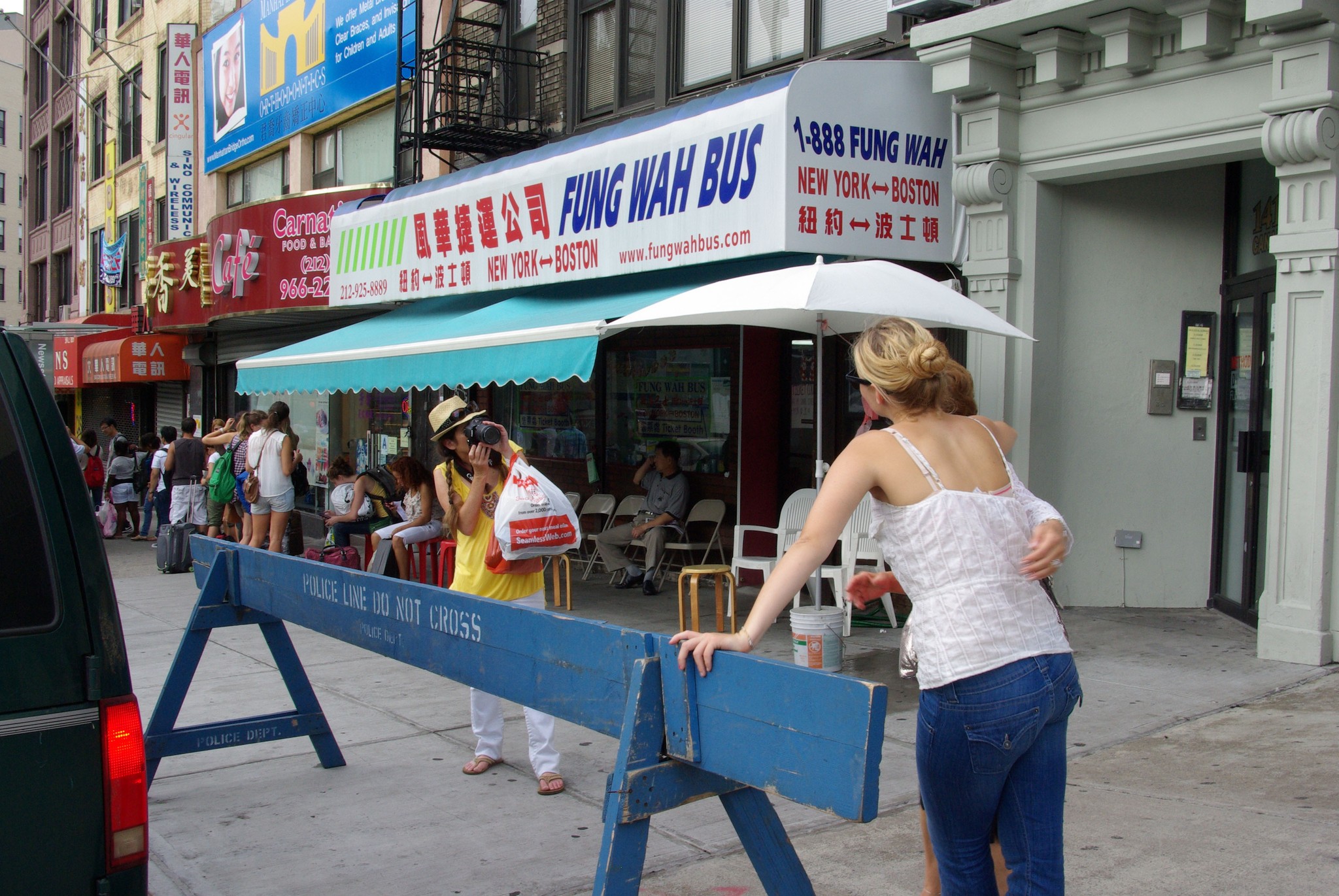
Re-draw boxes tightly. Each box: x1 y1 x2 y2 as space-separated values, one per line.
561 491 726 594
801 490 899 635
725 487 823 625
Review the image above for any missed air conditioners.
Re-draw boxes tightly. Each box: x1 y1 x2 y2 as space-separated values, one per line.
58 305 71 322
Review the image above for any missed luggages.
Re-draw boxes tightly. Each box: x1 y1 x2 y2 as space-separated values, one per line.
281 511 304 558
156 475 198 574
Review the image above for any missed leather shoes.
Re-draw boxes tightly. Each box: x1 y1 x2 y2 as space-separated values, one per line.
148 535 158 541
615 572 645 589
643 580 658 595
130 535 147 540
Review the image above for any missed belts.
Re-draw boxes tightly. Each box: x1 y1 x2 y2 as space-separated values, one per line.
637 510 661 516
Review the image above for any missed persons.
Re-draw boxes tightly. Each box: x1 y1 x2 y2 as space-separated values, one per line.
594 440 689 596
428 394 566 797
518 414 631 465
64 401 444 583
671 316 1085 895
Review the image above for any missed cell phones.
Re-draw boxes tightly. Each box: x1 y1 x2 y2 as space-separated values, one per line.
385 502 394 507
318 513 331 519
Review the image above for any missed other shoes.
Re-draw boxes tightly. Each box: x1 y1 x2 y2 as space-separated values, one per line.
127 531 140 537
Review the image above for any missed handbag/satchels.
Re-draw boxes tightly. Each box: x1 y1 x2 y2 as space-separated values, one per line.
306 544 362 571
98 499 110 526
493 456 581 562
243 465 261 504
484 452 544 574
103 501 118 539
290 451 309 497
132 469 148 494
331 483 376 522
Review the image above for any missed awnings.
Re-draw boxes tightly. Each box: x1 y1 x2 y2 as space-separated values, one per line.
329 58 970 308
47 313 189 387
234 255 850 396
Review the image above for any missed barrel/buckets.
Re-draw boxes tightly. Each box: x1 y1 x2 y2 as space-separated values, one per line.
790 606 847 672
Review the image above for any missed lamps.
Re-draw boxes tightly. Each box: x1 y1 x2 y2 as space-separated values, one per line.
94 28 107 47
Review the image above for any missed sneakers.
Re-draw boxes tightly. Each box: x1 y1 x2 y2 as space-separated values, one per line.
121 525 132 533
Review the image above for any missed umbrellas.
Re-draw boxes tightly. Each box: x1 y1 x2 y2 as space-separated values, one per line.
602 254 1040 613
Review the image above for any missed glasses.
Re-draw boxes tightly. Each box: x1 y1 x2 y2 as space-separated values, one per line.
101 425 111 431
430 400 479 437
845 367 871 390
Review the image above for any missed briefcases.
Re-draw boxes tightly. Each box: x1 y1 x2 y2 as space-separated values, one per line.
370 538 400 578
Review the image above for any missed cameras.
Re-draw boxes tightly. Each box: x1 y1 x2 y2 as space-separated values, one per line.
463 414 500 447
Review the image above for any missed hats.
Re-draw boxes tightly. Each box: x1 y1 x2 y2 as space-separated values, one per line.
428 395 486 442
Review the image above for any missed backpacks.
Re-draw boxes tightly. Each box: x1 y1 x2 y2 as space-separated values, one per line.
81 444 105 487
208 434 246 504
355 464 405 517
156 448 175 491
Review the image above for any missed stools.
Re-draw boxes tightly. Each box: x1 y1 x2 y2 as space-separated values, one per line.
437 539 459 589
407 537 448 586
539 553 573 611
674 562 737 637
351 532 375 572
